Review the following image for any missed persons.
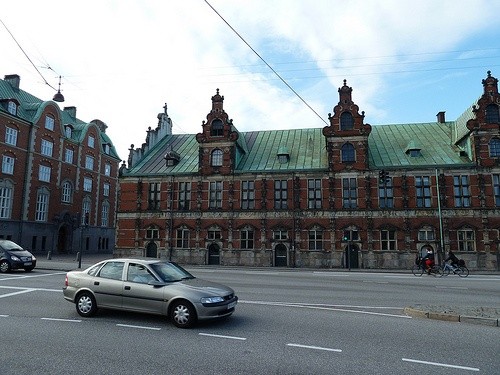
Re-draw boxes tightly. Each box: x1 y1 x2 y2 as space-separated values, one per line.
421 248 435 272
443 251 460 271
133 266 150 284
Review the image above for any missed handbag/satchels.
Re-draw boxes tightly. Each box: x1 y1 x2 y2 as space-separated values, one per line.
458 260 465 266
415 257 420 265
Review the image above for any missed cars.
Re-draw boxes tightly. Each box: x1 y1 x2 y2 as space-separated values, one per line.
62 257 238 329
0 239 37 274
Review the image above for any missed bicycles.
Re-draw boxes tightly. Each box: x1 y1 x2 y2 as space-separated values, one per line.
438 259 470 278
411 256 444 278
